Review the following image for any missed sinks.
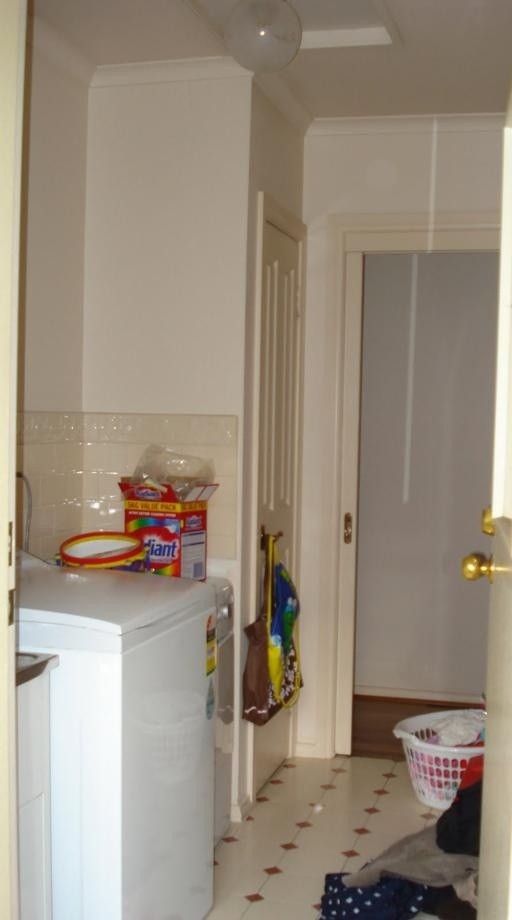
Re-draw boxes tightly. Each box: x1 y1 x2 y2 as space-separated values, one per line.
17 649 58 690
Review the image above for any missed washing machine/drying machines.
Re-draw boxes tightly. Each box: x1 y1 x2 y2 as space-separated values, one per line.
206 575 233 847
18 544 216 880
